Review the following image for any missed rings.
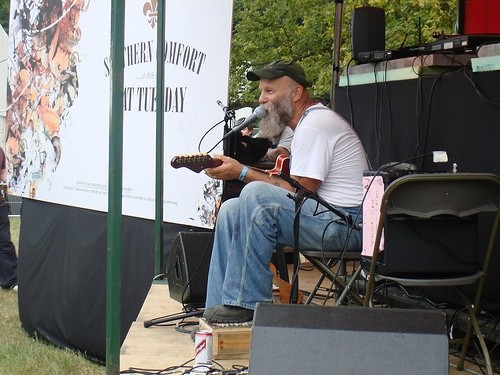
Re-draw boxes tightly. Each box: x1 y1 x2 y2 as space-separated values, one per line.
214 176 217 179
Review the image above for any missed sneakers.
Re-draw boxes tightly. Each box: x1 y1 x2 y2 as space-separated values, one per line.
203 304 254 323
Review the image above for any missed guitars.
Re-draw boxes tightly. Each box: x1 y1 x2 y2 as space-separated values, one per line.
170 154 291 181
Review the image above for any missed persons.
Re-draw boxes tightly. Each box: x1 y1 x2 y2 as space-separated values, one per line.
235 115 293 163
0 149 23 292
191 59 369 343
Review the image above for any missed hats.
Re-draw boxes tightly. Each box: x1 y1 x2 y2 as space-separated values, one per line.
246 59 312 90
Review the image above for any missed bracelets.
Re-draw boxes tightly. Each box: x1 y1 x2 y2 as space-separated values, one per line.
238 165 249 181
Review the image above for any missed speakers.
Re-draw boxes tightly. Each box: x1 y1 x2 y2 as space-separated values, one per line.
360 171 480 279
248 303 449 375
351 7 386 63
166 231 215 303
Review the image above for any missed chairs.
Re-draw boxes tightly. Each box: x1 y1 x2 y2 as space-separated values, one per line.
300 163 419 306
363 172 500 375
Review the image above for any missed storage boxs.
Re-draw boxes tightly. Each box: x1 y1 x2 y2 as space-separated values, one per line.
198 317 251 360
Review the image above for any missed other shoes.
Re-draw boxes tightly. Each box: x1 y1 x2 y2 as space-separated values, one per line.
9 284 18 291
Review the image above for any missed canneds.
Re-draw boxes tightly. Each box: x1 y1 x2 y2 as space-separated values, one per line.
195 330 213 365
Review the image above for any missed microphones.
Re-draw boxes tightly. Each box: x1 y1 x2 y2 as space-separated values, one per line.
223 106 266 140
335 220 363 230
